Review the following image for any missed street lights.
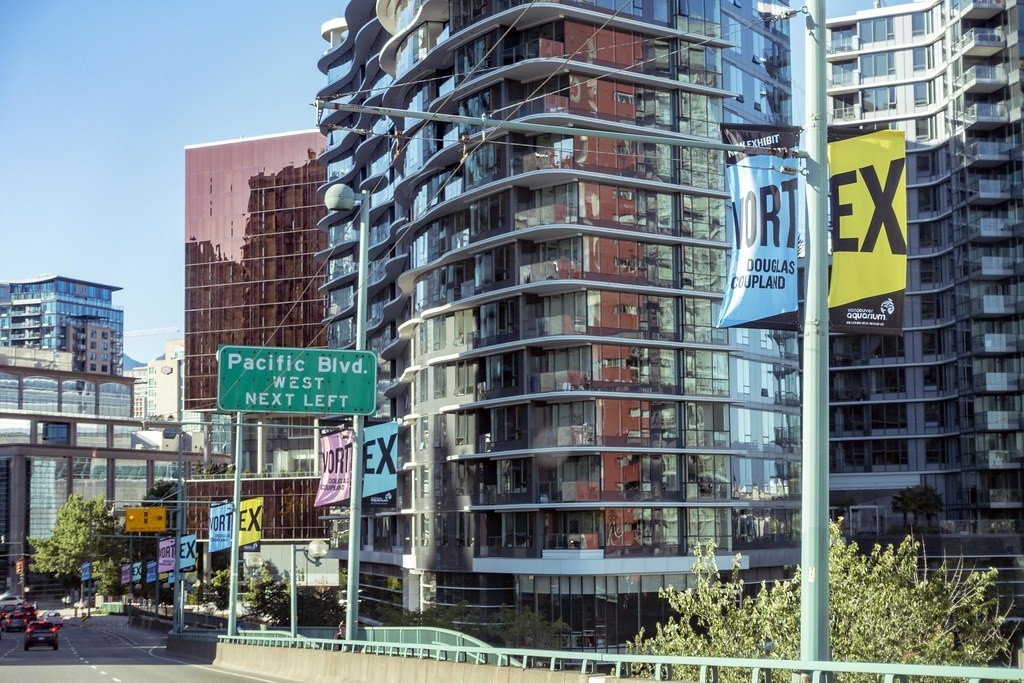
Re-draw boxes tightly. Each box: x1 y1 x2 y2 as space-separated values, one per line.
323 182 371 650
163 427 183 634
83 504 92 618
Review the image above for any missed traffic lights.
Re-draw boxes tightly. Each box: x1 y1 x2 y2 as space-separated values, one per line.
16 561 23 575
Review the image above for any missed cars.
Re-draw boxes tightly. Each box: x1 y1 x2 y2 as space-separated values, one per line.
0 592 39 634
39 610 64 627
24 619 59 650
